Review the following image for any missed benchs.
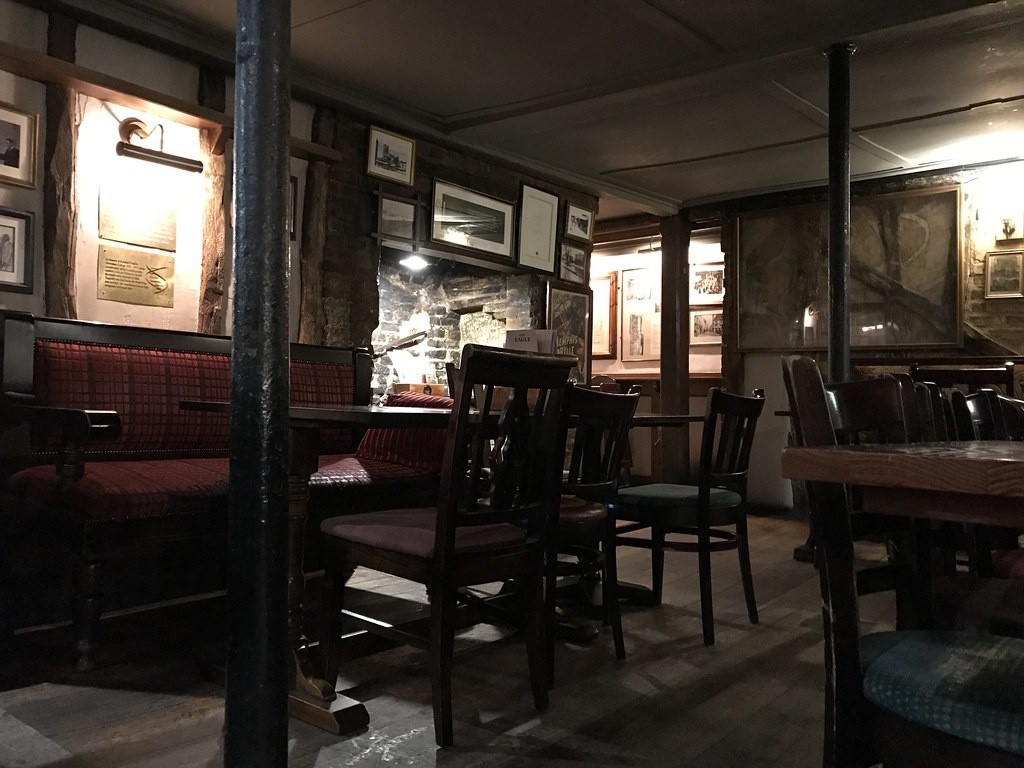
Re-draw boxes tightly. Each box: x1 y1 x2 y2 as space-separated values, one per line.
0 309 456 672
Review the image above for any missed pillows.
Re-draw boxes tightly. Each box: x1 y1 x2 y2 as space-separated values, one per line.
354 392 455 469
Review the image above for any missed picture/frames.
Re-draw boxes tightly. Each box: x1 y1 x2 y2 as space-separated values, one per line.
621 267 662 361
430 177 517 260
0 206 35 295
0 99 40 190
591 270 618 361
984 251 1024 299
688 261 724 308
367 125 416 188
564 202 594 245
558 242 586 286
230 159 297 241
516 181 561 277
545 278 593 388
689 308 723 347
731 183 964 354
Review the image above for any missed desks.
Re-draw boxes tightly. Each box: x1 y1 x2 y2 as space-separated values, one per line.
780 440 1023 638
179 400 706 736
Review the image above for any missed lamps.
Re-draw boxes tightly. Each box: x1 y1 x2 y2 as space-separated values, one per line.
115 118 203 174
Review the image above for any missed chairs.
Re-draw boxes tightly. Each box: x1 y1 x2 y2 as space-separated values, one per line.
321 343 767 747
780 355 1024 768
